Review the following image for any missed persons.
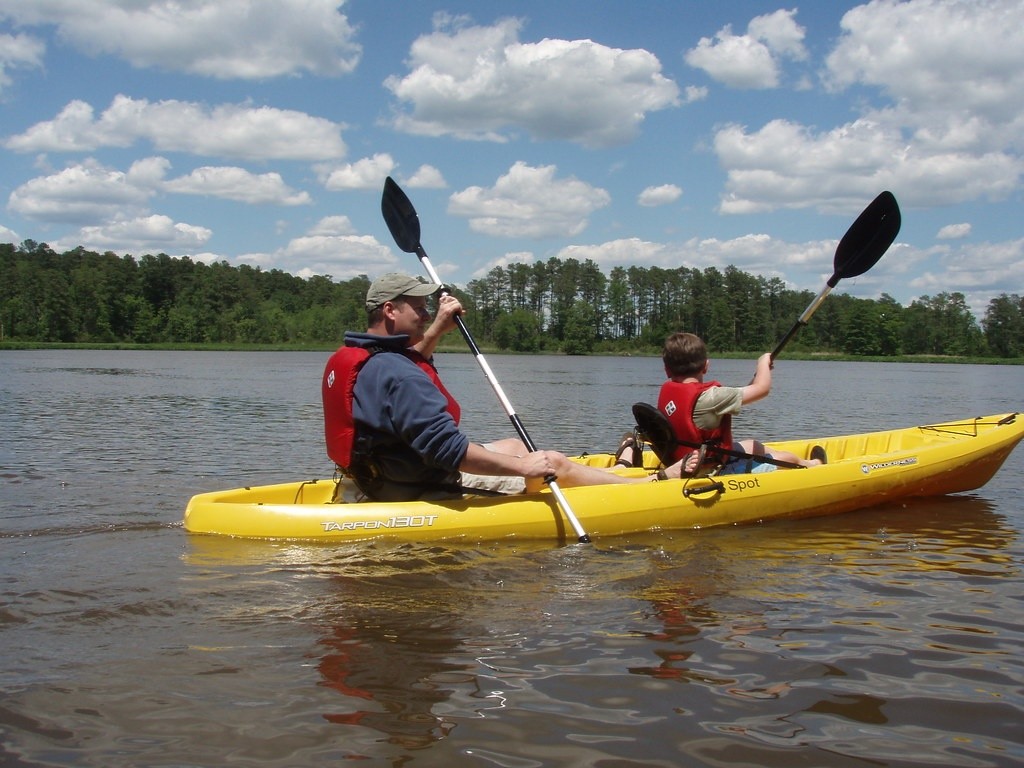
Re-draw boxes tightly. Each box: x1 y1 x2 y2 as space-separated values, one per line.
658 333 827 479
322 273 706 504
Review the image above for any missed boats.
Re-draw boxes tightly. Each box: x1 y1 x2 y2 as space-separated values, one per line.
179 410 1024 541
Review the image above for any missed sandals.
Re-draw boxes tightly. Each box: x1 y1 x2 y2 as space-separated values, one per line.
614 432 637 468
656 449 704 480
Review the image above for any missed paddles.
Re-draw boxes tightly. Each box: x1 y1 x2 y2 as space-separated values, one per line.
748 189 904 385
380 175 593 547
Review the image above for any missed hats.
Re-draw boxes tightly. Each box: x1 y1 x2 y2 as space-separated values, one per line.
366 273 441 314
